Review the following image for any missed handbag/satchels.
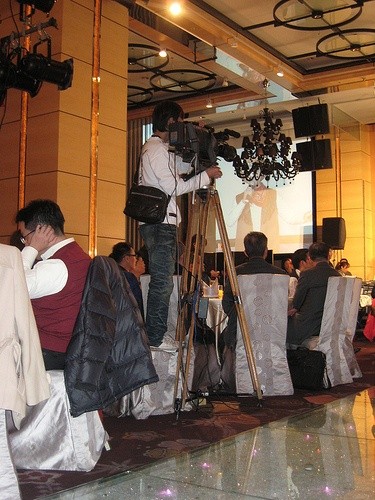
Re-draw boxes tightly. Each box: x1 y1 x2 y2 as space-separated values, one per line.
286 346 332 390
123 185 166 223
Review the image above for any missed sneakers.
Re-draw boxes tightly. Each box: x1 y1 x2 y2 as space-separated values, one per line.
149 339 186 352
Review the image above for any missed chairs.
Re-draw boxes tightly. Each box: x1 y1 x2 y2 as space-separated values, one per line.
10 255 363 476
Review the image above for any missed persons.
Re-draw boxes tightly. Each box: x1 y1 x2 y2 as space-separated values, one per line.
234 147 303 249
15 199 92 372
137 101 221 351
110 232 352 398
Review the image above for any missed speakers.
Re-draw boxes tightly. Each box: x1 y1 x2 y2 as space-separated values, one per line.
292 103 330 137
296 138 332 172
322 217 346 250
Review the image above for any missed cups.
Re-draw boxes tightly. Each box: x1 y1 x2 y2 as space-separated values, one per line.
210 281 214 286
218 285 223 299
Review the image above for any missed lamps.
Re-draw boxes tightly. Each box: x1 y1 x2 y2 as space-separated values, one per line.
160 44 165 56
206 98 213 108
242 112 247 121
229 79 300 187
23 38 74 91
17 0 56 14
0 48 42 99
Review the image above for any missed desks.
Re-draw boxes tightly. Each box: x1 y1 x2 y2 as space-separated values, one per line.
206 296 227 330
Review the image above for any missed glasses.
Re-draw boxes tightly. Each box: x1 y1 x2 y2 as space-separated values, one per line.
20 228 41 244
128 253 137 257
